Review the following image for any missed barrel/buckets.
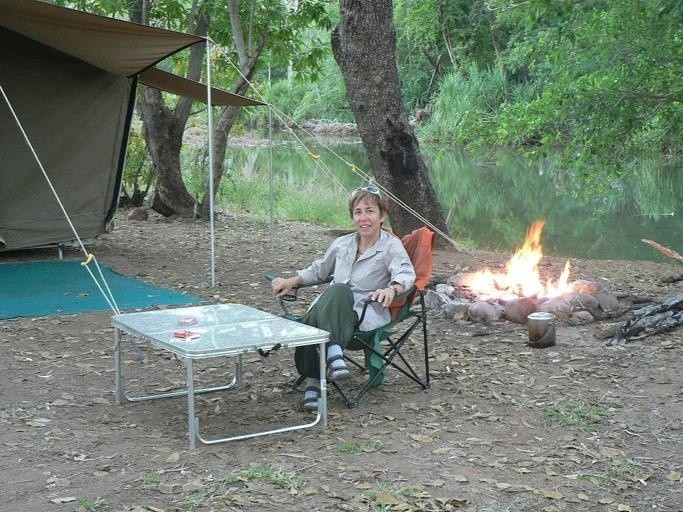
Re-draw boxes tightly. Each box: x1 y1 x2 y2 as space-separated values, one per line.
529 312 556 348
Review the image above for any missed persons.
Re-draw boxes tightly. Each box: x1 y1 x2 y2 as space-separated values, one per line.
271 186 416 410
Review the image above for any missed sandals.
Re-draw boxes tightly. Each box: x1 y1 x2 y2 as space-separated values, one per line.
303 386 321 412
327 356 351 382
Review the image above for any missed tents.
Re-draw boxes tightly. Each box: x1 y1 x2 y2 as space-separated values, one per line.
0 0 269 252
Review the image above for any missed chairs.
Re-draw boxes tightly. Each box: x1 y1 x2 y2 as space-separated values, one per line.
263 227 430 408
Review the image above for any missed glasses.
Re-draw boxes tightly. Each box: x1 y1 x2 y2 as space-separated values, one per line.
352 186 382 200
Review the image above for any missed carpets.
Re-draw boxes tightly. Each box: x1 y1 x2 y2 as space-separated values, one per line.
1 260 208 322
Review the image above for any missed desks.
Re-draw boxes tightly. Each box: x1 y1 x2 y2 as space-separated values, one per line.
109 302 331 451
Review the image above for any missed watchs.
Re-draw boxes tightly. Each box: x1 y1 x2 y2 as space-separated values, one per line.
388 284 398 297
